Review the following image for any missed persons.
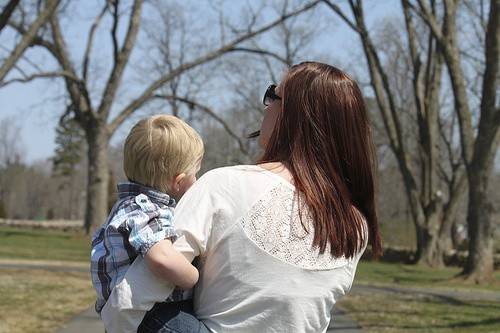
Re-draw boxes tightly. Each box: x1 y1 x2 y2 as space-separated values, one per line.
91 114 212 333
100 60 383 333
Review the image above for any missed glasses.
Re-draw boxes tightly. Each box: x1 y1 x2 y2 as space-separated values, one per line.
263 85 282 106
